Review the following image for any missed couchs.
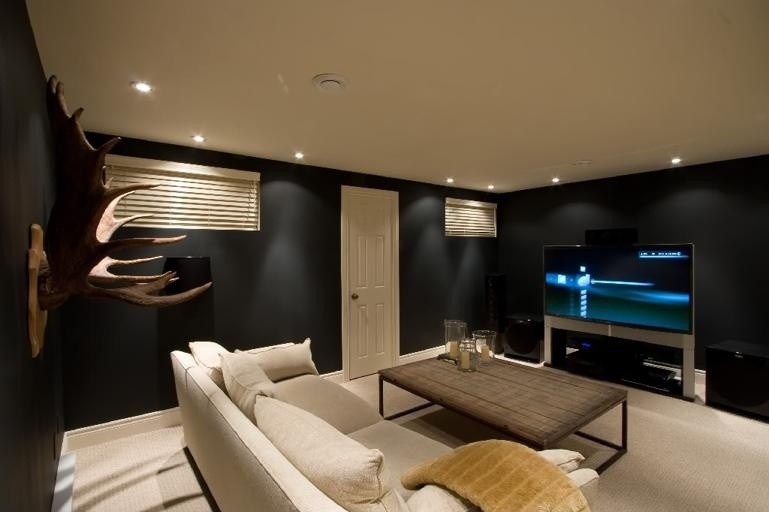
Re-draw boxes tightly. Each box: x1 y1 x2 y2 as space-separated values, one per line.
169 342 599 512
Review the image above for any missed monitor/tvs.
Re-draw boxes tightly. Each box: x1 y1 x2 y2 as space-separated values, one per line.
541 241 695 335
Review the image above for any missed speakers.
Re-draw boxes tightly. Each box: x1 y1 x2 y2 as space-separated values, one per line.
704 340 769 424
502 311 546 364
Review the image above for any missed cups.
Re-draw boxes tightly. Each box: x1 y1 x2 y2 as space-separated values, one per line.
444 319 467 360
473 330 497 366
455 339 478 373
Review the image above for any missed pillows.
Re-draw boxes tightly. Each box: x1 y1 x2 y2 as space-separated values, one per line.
189 340 230 394
218 352 282 427
254 394 400 512
234 337 319 381
